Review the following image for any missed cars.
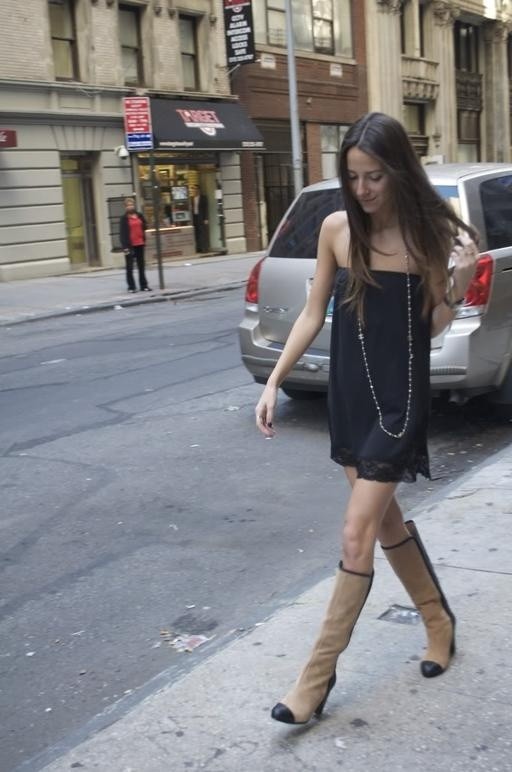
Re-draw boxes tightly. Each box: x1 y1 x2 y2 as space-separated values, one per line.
240 161 512 417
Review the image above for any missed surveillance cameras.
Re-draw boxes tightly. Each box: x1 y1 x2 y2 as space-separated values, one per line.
118 146 129 160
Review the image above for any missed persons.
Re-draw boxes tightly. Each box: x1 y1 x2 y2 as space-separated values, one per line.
254 112 480 725
120 197 152 293
189 185 209 253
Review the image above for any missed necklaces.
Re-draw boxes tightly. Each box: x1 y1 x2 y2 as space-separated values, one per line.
356 243 414 438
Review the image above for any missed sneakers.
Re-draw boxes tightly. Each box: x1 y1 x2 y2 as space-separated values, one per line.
128 288 137 293
140 287 151 291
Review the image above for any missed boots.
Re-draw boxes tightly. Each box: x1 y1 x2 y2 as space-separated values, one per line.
271 560 374 726
381 520 456 677
